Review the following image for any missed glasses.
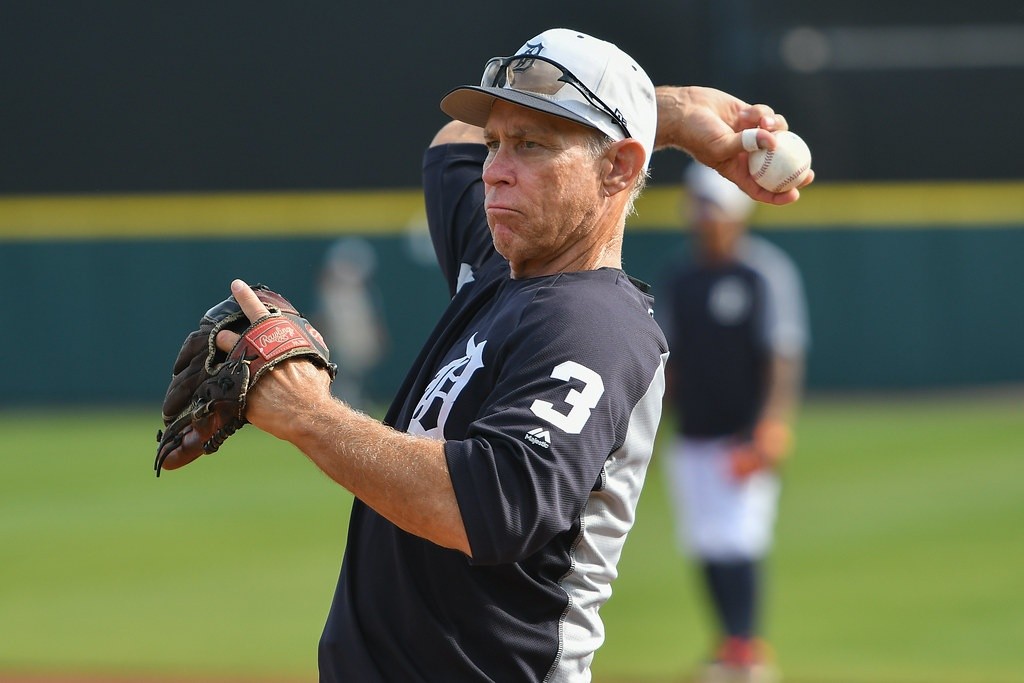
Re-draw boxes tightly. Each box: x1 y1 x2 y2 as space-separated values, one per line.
480 54 632 138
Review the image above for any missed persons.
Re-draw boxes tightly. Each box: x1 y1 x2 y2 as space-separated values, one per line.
158 28 815 683
647 158 811 683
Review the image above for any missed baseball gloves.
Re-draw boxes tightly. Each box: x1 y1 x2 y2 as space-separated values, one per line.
154 283 338 478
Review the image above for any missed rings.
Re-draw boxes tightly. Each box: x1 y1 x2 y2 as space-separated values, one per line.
741 129 761 154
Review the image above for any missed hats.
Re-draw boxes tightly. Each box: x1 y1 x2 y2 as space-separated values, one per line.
683 161 753 220
440 28 658 177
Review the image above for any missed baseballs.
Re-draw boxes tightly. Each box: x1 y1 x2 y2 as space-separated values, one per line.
748 129 814 194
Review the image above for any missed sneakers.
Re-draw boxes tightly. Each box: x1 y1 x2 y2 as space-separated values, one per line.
706 636 782 683
